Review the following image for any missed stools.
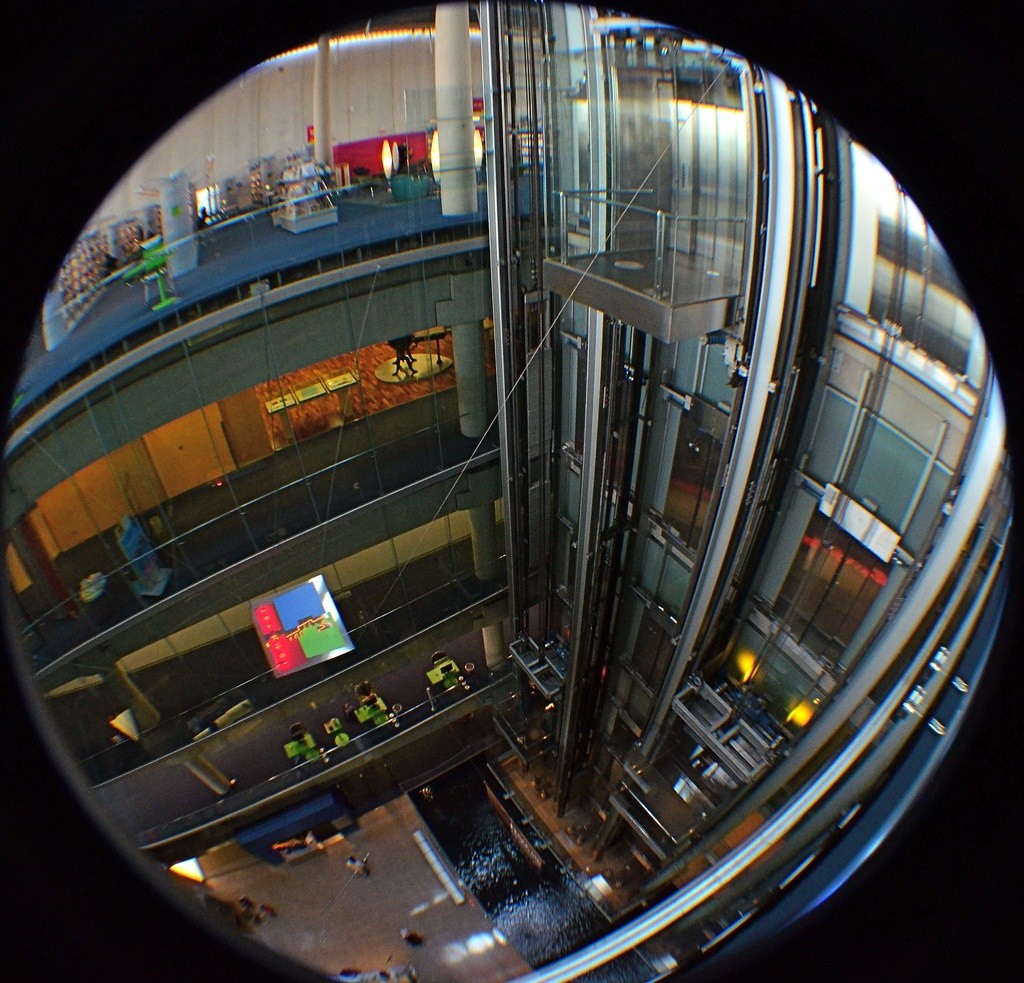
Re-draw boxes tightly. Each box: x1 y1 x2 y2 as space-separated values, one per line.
123 258 168 289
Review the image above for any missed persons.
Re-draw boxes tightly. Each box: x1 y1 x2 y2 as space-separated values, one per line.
260 904 275 917
400 930 421 947
347 853 370 876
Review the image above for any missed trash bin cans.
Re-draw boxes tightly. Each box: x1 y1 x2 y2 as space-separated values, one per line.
391 703 402 714
81 571 124 626
465 662 475 676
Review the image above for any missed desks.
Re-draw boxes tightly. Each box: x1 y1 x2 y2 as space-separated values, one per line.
284 734 319 763
354 698 388 726
426 660 459 689
144 242 177 310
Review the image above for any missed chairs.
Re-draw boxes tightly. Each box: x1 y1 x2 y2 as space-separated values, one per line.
324 718 349 747
430 650 447 669
290 722 307 735
